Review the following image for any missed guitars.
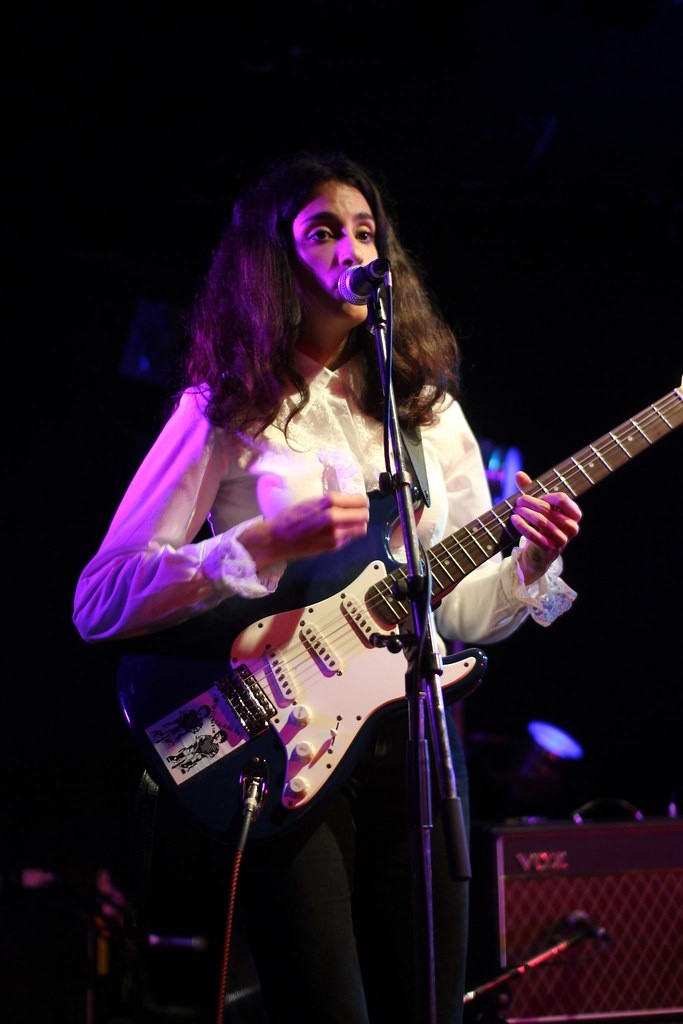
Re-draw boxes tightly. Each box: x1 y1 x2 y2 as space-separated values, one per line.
110 378 683 851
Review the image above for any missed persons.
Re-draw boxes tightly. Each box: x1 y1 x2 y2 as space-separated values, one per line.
71 156 582 1023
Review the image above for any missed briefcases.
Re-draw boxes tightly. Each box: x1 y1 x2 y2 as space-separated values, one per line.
490 797 683 1024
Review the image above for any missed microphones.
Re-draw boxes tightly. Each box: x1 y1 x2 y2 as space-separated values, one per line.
336 257 390 306
